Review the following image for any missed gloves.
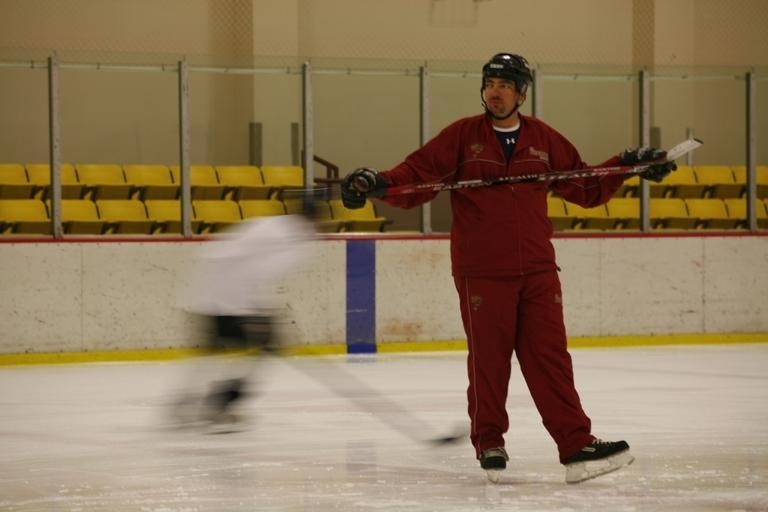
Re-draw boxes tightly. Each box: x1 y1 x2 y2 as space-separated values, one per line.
620 146 677 181
341 167 387 208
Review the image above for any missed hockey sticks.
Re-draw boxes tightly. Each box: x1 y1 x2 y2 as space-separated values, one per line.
382 136 702 199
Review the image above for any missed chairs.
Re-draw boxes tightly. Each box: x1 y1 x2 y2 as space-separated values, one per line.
24 165 102 231
1 163 50 234
546 163 768 230
215 165 286 227
76 165 152 233
121 164 197 232
170 164 243 229
261 164 333 228
329 197 388 232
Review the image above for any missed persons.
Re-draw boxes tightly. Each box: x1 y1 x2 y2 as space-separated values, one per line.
341 52 676 473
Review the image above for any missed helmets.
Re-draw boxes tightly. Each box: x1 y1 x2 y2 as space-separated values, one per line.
483 52 533 92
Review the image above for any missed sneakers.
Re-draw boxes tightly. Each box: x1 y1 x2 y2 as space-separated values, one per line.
479 446 506 469
561 439 629 465
